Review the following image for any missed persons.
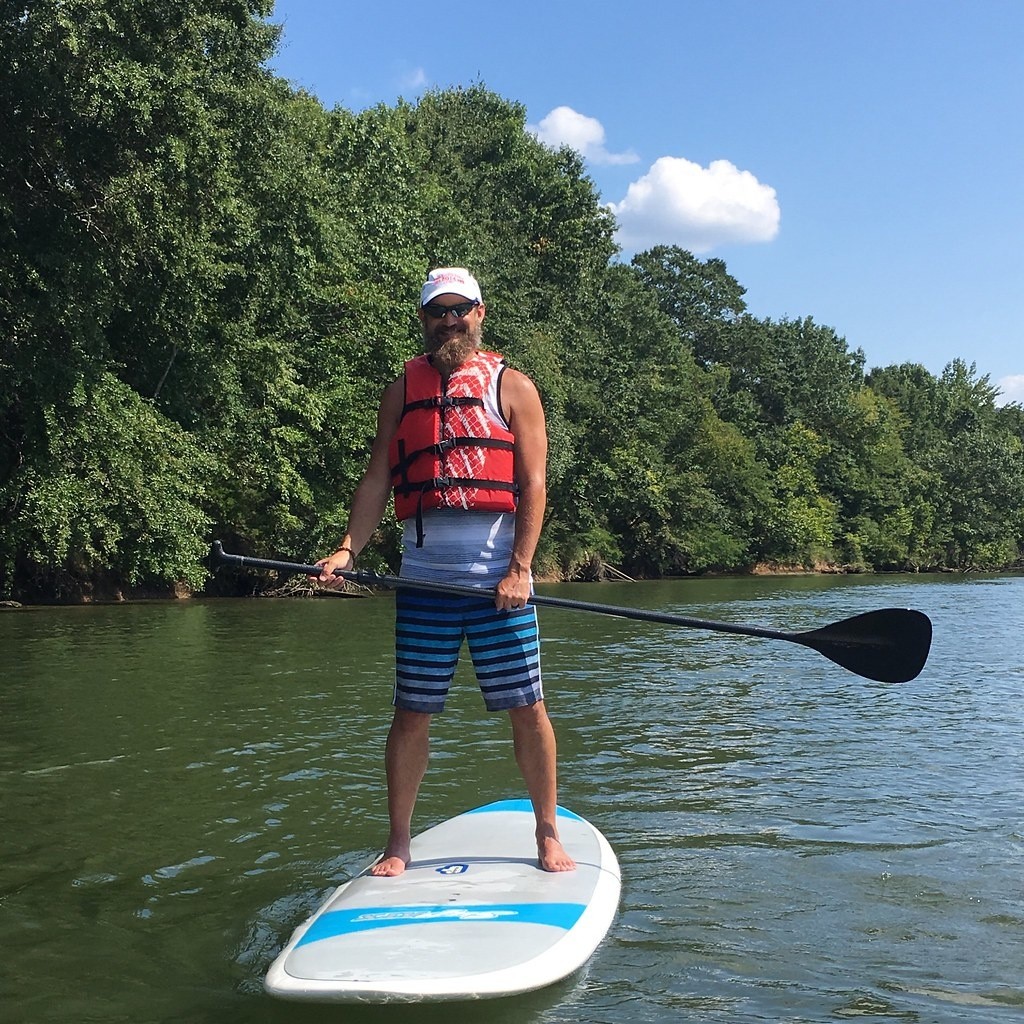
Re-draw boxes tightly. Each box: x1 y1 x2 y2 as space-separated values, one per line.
307 267 577 877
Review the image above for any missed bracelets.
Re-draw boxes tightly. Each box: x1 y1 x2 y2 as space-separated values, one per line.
334 547 357 567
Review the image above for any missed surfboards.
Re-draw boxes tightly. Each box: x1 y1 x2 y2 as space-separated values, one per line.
262 799 624 999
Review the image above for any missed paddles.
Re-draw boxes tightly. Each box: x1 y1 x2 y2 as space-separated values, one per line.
213 537 933 684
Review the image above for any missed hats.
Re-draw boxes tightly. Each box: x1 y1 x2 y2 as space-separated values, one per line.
421 268 483 306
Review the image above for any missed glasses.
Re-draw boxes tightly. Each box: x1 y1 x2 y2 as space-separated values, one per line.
422 301 479 318
